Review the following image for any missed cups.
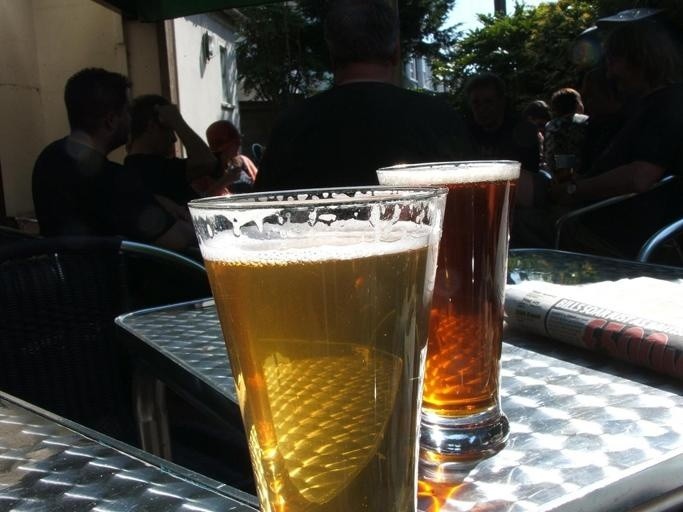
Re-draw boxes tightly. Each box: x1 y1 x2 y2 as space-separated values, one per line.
376 159 521 455
187 186 448 512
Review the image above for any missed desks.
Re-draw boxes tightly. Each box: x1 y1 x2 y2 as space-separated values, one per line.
0 392 268 511
114 247 683 511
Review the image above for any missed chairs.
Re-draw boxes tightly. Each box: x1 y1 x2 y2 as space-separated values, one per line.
0 217 44 244
0 237 211 449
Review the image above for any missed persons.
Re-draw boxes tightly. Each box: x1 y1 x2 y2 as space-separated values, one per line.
115 94 227 268
253 0 469 193
31 66 213 334
466 21 682 263
205 120 259 196
